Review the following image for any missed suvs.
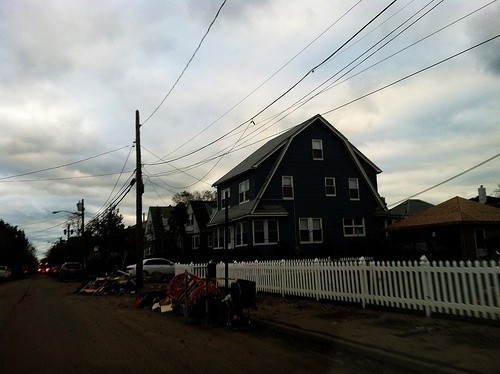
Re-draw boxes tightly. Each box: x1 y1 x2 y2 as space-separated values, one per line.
39 262 82 281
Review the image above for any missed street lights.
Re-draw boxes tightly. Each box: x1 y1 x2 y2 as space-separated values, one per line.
51 210 86 283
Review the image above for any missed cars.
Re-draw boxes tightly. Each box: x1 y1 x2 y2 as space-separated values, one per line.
126 258 176 277
0 265 12 279
